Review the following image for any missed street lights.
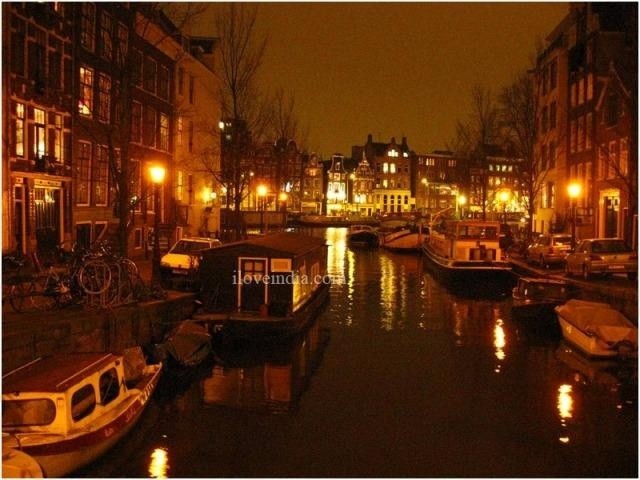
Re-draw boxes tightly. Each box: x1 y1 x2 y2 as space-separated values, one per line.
278 191 289 232
458 195 468 220
498 189 513 225
568 182 582 252
146 162 169 301
257 185 268 236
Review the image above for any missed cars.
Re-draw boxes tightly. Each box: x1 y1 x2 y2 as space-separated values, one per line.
159 235 224 293
382 210 422 219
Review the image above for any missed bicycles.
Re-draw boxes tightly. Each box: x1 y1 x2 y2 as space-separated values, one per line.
1 235 138 314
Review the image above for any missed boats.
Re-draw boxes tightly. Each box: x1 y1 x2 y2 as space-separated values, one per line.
346 217 639 363
3 343 165 476
192 230 332 329
191 313 332 420
